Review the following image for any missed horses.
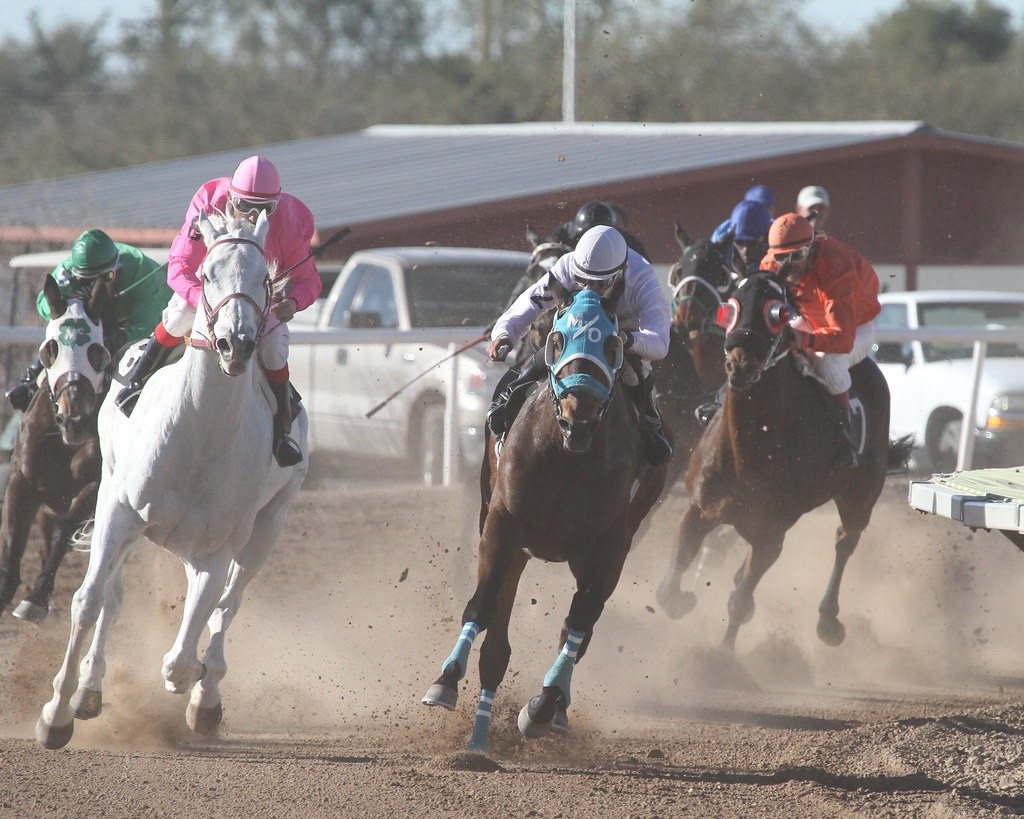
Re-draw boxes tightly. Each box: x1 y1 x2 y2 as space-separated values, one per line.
34 204 312 751
648 220 917 657
422 213 673 768
1 272 120 625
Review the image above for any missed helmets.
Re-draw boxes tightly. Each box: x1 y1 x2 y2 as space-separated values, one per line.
575 200 617 234
230 155 281 204
744 184 775 208
71 230 120 278
571 224 629 279
729 200 772 240
765 212 816 254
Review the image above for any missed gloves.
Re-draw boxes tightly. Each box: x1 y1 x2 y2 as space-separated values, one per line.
487 337 513 362
269 299 297 324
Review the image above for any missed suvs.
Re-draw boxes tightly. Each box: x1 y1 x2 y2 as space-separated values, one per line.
867 289 1024 472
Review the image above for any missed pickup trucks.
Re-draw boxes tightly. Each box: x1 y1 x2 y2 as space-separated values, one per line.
286 245 533 485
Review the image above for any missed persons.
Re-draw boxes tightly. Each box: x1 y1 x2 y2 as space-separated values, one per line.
758 213 882 446
485 224 676 466
10 229 174 412
115 155 322 467
548 199 652 265
714 184 775 279
796 186 829 230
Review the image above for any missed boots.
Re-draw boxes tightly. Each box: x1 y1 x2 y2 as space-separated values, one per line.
834 405 858 469
625 373 672 469
487 345 552 435
114 331 178 419
267 378 302 467
5 357 45 412
695 380 727 426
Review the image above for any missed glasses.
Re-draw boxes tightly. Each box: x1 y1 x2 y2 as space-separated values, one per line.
733 237 762 249
573 273 617 290
77 270 116 290
231 196 278 216
773 247 810 264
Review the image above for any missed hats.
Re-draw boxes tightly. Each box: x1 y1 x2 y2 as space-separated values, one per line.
797 186 830 209
